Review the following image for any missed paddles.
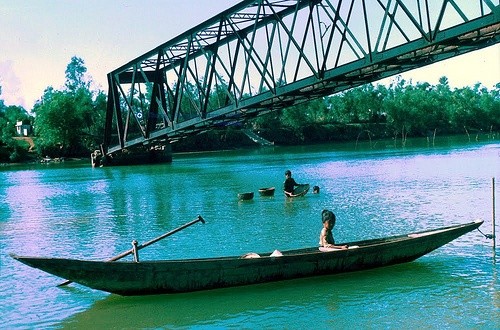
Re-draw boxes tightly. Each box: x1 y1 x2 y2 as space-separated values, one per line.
55 215 206 287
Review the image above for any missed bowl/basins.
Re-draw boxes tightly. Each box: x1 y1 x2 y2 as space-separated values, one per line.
258 187 275 196
238 192 254 200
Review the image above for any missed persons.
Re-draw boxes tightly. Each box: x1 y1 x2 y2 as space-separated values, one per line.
283 170 301 198
311 186 320 194
318 209 347 249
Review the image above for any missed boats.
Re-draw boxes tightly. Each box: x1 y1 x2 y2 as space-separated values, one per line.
10 219 483 297
